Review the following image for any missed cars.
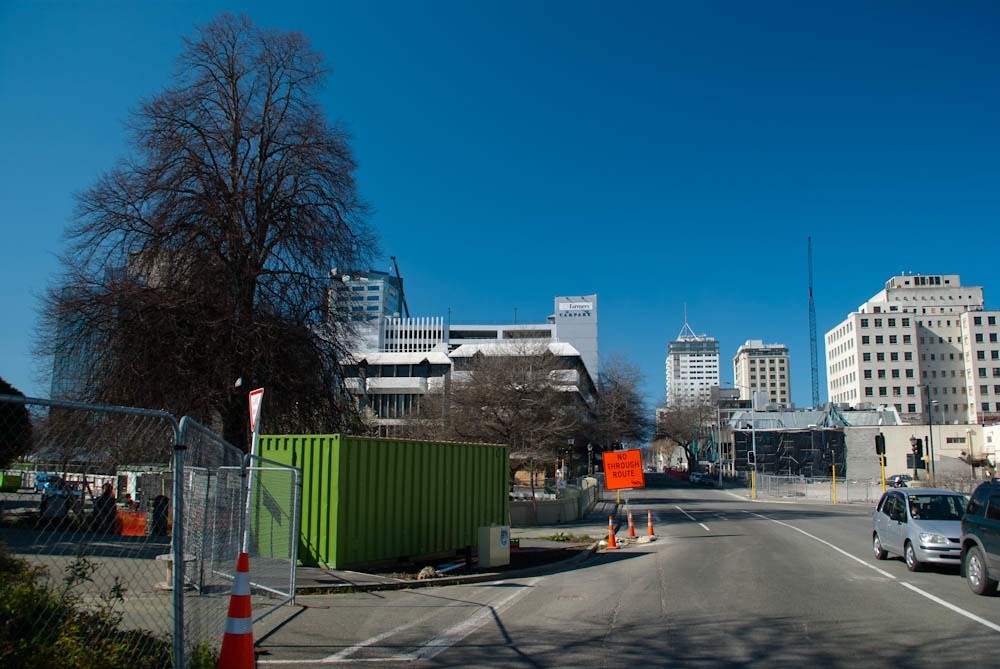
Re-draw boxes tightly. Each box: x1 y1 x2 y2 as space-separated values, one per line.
871 488 969 571
878 474 913 487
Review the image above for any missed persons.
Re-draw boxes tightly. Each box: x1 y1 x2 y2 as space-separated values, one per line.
910 505 922 519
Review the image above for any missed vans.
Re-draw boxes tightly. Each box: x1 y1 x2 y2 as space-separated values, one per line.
690 473 709 484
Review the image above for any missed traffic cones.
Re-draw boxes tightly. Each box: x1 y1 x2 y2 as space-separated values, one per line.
646 510 656 536
606 516 620 549
627 511 638 539
215 552 256 669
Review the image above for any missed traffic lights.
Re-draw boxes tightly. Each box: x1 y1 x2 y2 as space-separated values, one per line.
912 439 923 458
879 456 886 467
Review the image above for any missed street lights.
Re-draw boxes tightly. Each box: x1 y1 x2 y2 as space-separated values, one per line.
928 400 940 488
587 444 592 473
568 434 575 482
910 436 918 480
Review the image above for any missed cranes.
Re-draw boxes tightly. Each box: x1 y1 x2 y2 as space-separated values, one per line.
806 236 819 408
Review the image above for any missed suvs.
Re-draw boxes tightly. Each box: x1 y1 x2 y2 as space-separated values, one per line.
41 481 83 502
960 477 1000 595
33 473 61 494
